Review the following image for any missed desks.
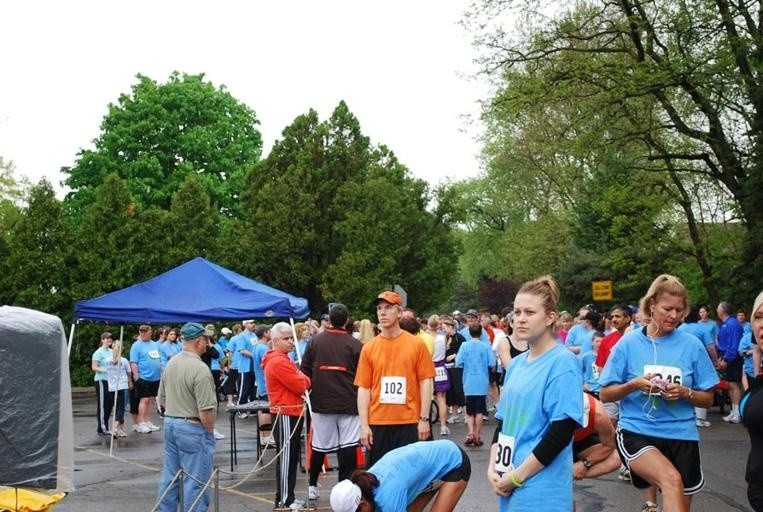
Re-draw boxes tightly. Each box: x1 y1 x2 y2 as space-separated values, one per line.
227 398 271 476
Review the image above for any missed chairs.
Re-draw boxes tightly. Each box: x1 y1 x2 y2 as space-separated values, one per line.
256 410 277 467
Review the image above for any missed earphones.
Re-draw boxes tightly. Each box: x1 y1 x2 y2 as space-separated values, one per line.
651 305 655 311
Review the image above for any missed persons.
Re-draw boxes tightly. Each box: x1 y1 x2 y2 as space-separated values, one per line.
329 439 471 512
739 292 763 511
299 303 364 500
153 322 219 511
596 271 721 511
260 321 312 511
574 392 621 479
352 290 436 470
487 272 584 512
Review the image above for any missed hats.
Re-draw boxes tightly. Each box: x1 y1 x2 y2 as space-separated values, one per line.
139 319 273 340
100 331 114 339
579 312 603 323
328 477 362 512
372 290 403 306
320 313 330 321
453 309 463 316
465 309 478 317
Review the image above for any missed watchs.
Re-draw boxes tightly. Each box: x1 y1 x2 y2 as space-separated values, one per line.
581 458 592 470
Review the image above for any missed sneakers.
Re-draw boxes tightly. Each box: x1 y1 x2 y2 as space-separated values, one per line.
273 501 304 511
720 409 734 422
113 419 161 438
439 404 491 436
696 417 712 428
729 411 742 424
214 402 277 450
307 485 321 501
617 465 633 481
463 432 476 445
474 439 484 447
641 504 660 512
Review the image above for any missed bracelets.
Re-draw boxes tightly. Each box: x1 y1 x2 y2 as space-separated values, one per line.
511 469 526 488
